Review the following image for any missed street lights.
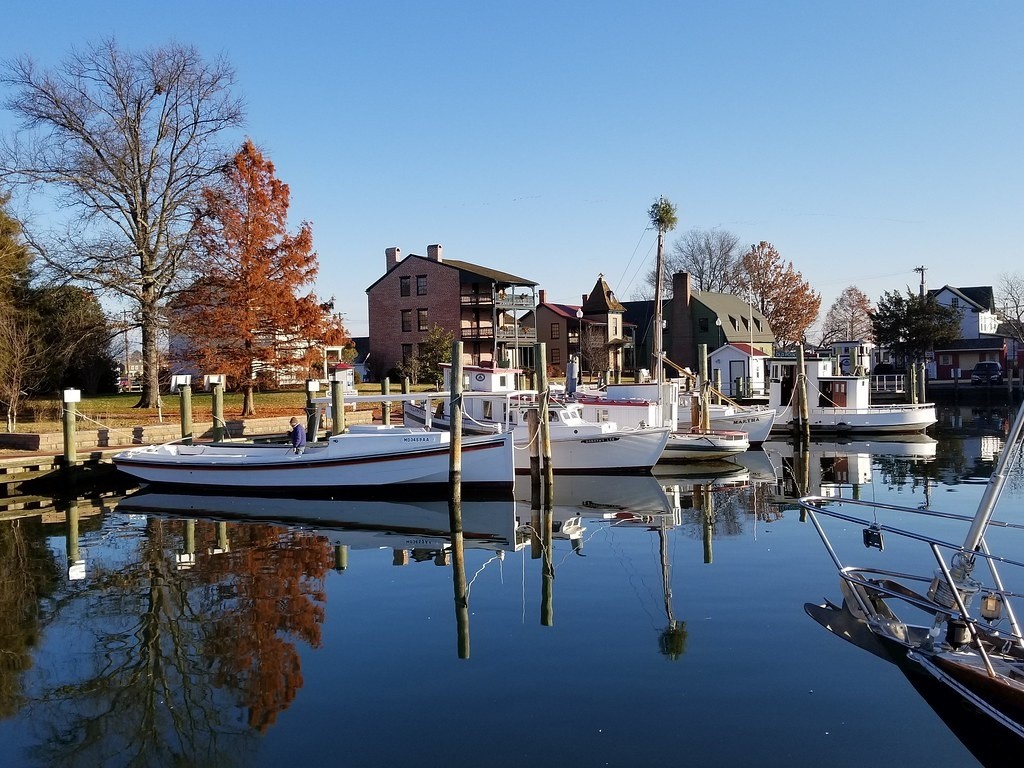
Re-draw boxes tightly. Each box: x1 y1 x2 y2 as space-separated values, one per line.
714 315 725 347
575 307 586 384
914 266 925 307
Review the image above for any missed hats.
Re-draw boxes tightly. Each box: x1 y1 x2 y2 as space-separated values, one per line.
290 417 298 423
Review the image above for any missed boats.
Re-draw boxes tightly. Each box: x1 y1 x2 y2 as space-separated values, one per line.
111 481 587 553
689 433 947 520
649 458 753 485
110 353 940 498
515 476 678 522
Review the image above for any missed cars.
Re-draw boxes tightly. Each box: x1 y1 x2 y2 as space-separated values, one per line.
969 360 1006 387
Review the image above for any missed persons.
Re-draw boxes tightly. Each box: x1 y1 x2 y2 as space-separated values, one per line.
286 417 306 455
